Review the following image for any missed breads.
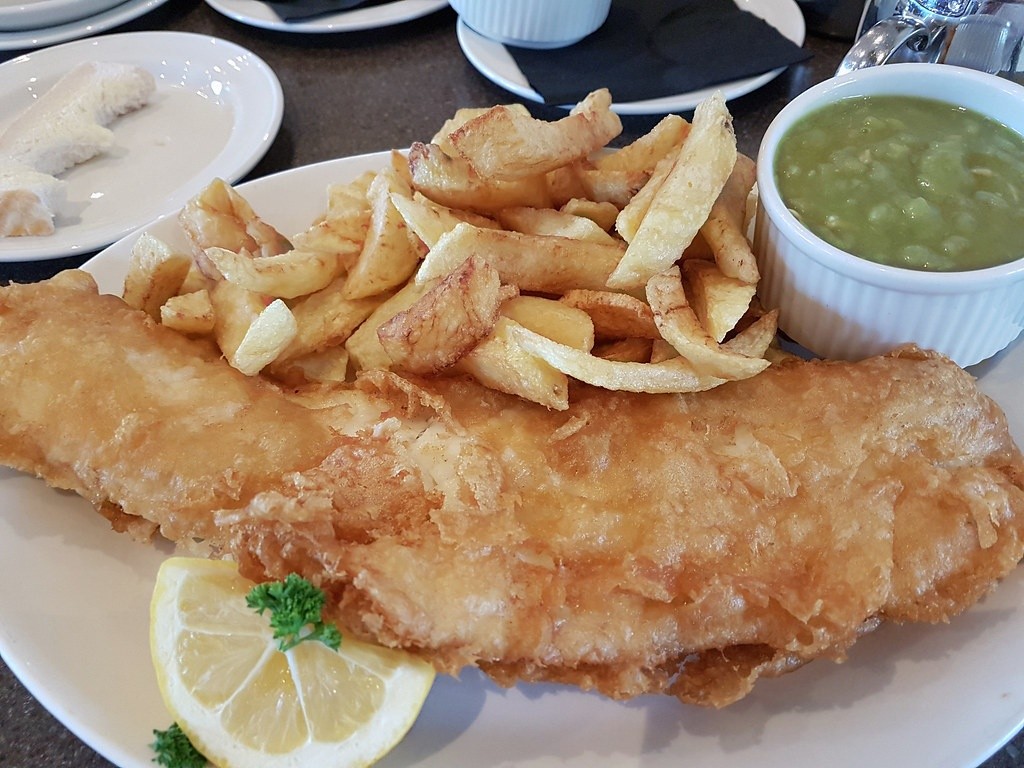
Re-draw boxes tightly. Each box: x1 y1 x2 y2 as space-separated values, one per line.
0 60 153 239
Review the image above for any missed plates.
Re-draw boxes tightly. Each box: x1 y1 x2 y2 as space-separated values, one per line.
0 1 169 52
0 31 285 267
0 137 1024 768
203 0 451 34
455 1 807 114
0 0 125 33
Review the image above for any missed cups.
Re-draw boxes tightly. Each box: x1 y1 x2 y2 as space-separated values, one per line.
756 65 1024 378
445 0 613 51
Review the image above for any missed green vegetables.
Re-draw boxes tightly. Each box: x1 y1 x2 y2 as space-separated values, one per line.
148 572 342 767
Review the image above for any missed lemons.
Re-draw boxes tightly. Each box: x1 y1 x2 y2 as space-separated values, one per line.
148 556 437 768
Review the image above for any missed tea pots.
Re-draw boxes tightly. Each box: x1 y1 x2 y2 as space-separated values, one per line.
830 0 1024 87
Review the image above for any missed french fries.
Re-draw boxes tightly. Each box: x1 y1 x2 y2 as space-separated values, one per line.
125 86 779 415
